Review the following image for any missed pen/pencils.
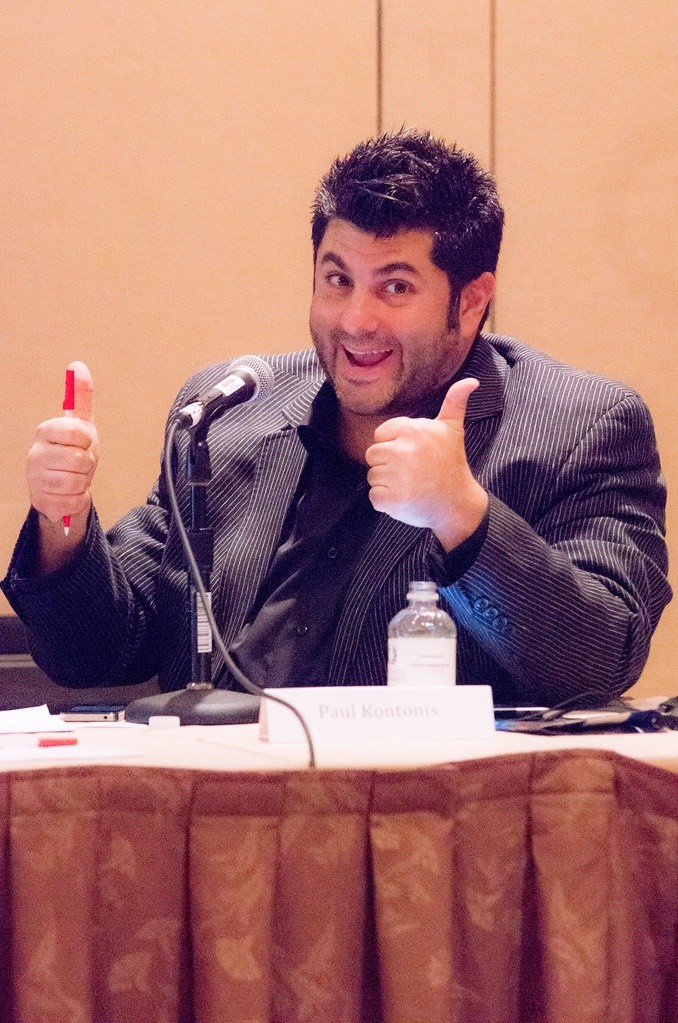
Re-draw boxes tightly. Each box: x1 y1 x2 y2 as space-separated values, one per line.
61 366 76 540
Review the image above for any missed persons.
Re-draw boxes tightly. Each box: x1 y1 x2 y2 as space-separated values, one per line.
0 124 673 715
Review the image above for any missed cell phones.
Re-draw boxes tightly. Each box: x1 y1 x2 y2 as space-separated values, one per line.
64 705 126 723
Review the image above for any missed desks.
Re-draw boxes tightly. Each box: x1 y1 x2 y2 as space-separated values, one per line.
0 698 678 1023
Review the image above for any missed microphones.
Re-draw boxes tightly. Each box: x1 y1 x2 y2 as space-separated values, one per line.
171 357 275 430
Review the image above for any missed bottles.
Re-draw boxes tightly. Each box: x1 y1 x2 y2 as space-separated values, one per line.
387 580 457 686
